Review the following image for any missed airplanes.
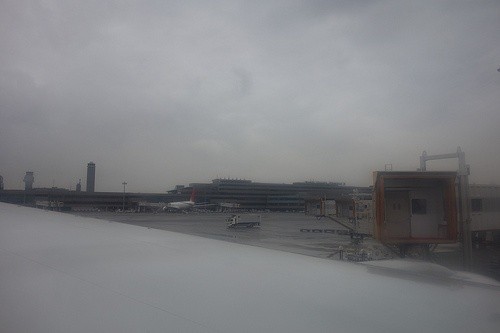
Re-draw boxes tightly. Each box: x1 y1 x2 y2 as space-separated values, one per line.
139 197 220 214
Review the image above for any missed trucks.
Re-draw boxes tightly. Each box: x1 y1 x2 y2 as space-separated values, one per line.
225 212 262 228
